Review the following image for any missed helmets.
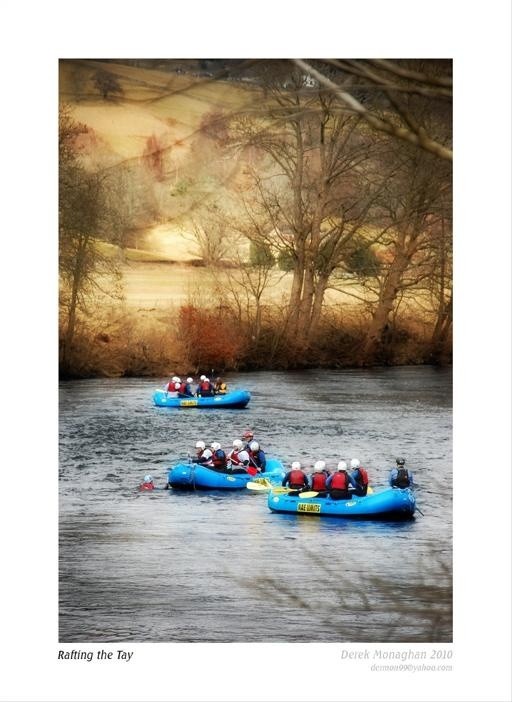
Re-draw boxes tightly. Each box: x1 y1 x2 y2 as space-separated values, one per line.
396 457 404 465
338 462 346 471
352 459 360 469
314 462 326 473
233 432 259 451
172 375 211 383
292 463 301 470
211 443 220 450
195 440 204 448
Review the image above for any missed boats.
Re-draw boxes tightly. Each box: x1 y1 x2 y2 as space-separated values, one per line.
168 458 286 489
152 388 252 408
268 474 414 516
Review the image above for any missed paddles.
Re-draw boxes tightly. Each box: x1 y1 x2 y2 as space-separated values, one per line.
271 487 302 495
247 482 274 491
298 491 329 498
249 455 273 488
229 457 257 476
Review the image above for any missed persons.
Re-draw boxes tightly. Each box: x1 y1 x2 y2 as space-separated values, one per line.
140 474 156 492
280 456 368 500
163 374 228 398
188 432 267 474
389 455 414 490
